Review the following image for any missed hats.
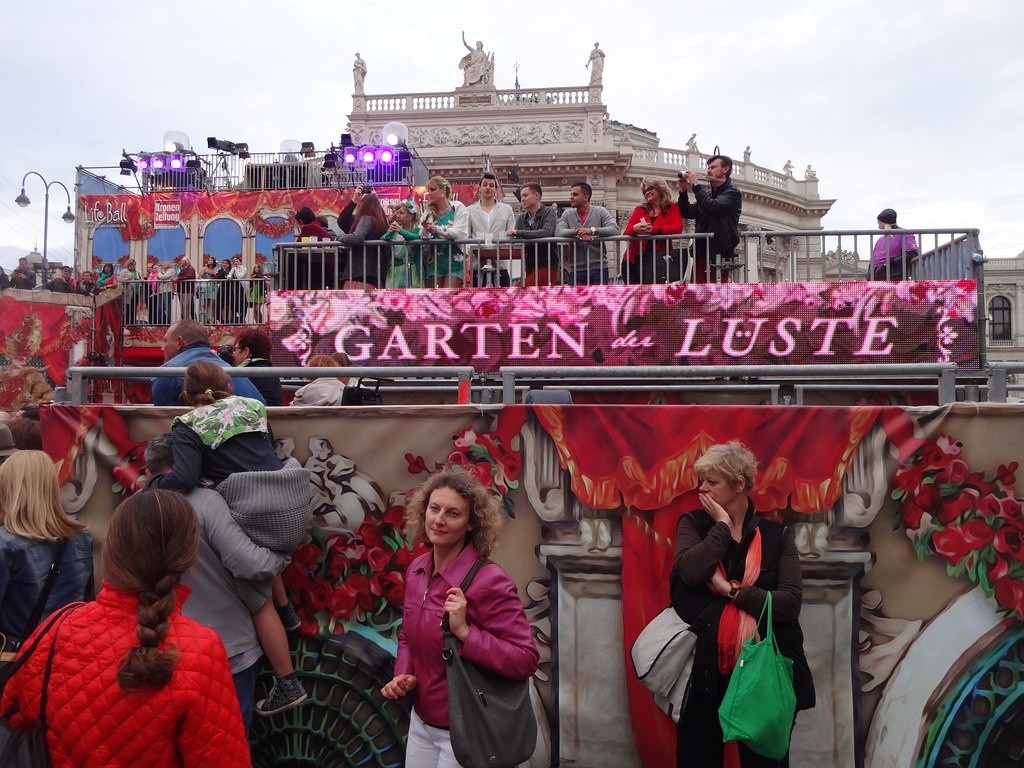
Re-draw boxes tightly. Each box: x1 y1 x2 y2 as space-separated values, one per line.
878 209 896 224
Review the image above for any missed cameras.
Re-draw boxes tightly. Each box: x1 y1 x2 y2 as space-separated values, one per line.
217 345 233 366
361 185 373 194
678 171 686 179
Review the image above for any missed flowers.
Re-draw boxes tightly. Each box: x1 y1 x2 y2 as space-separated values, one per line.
119 221 158 241
79 405 528 642
248 213 298 238
891 433 1023 628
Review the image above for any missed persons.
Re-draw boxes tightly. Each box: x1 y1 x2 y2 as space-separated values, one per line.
352 52 367 94
805 164 816 179
688 134 697 150
231 327 283 406
288 355 346 405
381 464 540 768
866 209 918 280
678 155 742 284
141 359 313 716
0 257 37 290
151 319 266 406
462 31 490 85
621 176 683 285
783 159 793 176
674 441 817 768
743 145 751 162
50 263 117 298
295 173 621 290
120 257 267 325
587 42 606 84
0 488 253 768
0 449 96 652
143 433 291 733
0 405 43 462
330 353 365 388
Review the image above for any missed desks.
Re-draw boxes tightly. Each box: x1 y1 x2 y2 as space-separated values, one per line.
469 243 522 289
281 247 347 290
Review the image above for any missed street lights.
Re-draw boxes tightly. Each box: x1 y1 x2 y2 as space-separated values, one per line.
13 170 75 290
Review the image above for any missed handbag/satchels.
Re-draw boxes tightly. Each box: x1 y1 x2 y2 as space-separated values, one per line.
719 591 797 759
441 633 537 768
629 604 699 724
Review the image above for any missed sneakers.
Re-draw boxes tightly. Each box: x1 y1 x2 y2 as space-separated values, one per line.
277 602 301 630
255 671 308 715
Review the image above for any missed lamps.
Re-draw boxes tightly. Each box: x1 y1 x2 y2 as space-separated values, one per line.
120 160 134 172
136 142 200 173
341 133 410 167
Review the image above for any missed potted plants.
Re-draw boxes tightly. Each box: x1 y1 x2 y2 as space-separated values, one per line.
323 153 337 168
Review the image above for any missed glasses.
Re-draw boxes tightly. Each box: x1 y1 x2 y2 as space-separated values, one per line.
643 187 654 195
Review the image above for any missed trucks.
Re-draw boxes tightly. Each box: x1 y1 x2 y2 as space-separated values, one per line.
0 118 1022 768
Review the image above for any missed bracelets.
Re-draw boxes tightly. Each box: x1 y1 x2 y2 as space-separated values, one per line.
591 227 595 235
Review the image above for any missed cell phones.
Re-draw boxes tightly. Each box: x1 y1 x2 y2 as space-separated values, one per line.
640 217 647 225
